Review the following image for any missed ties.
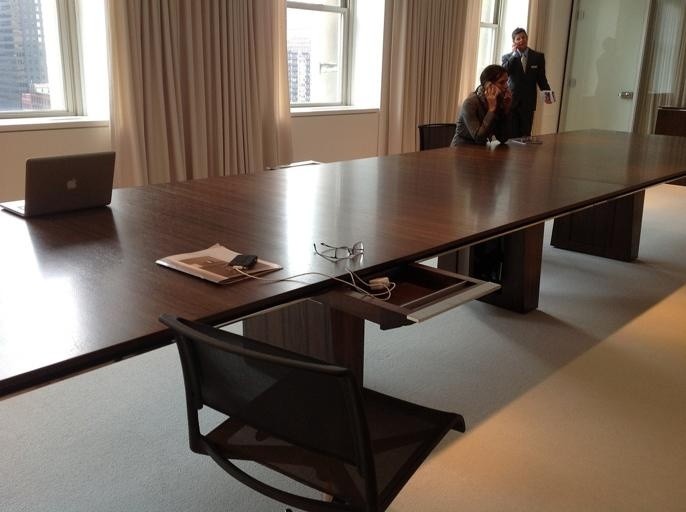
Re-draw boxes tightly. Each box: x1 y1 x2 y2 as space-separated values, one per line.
521 53 527 73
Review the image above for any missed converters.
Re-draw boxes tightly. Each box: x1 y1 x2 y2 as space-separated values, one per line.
369 276 390 290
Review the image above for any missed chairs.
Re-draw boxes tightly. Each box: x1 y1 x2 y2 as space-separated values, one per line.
654 106 686 135
156 314 467 512
417 123 457 151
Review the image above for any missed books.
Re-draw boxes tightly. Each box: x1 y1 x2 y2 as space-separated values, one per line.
155 241 284 286
540 90 556 104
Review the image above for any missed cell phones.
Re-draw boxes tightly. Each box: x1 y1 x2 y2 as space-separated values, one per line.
484 82 500 98
227 255 258 272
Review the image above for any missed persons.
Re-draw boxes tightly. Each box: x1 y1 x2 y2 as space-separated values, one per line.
501 27 551 137
449 64 514 147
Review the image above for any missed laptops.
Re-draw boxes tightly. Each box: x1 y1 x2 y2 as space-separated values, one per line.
0 151 117 217
